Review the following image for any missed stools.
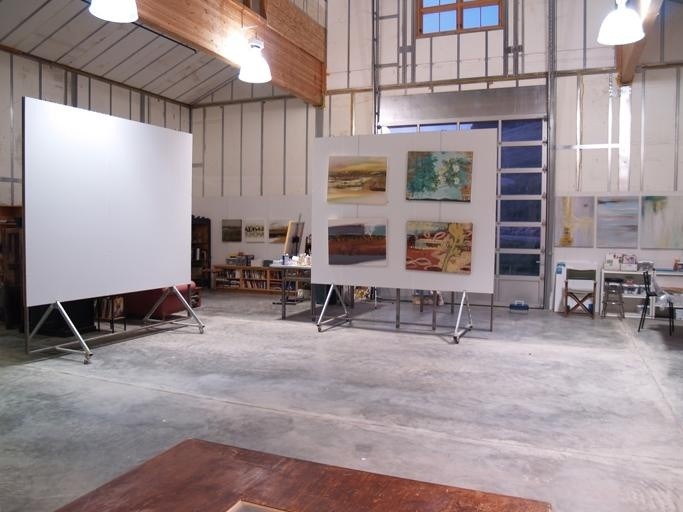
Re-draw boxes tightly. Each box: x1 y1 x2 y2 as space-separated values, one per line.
602 278 625 319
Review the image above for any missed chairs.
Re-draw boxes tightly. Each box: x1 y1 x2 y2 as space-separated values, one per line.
563 269 597 319
637 270 675 335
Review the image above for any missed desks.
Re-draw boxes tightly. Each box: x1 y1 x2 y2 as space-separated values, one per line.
271 263 314 318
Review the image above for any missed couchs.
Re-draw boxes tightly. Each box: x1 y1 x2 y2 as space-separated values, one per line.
126 281 198 312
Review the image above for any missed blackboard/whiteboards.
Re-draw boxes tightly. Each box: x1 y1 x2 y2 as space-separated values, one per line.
24 95 192 308
311 128 497 295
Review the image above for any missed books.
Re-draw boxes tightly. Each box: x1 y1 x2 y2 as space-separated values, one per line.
215 268 282 291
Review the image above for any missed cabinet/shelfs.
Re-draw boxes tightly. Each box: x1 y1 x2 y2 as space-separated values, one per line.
211 264 313 297
600 271 656 318
656 268 683 289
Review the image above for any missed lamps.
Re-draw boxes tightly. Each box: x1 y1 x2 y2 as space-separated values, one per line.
234 5 273 85
596 2 646 47
89 0 138 23
